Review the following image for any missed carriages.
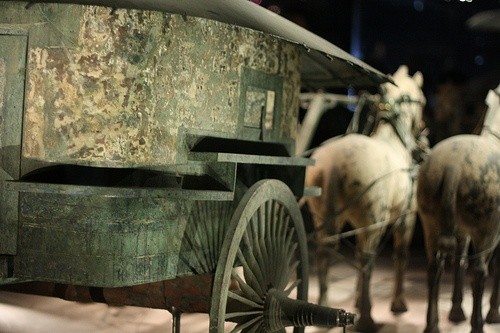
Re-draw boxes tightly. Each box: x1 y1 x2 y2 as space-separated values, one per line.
1 0 499 332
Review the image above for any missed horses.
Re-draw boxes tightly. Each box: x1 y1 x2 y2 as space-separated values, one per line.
304 65 430 333
415 84 500 333
